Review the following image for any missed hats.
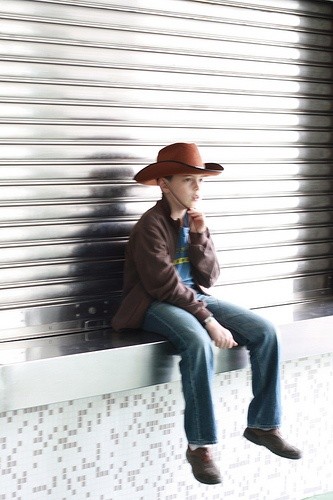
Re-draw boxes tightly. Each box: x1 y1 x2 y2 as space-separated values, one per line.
133 143 225 185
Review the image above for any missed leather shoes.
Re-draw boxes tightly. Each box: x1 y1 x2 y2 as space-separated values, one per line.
185 445 223 485
243 428 302 459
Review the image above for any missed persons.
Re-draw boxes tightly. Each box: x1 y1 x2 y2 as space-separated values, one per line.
111 143 302 485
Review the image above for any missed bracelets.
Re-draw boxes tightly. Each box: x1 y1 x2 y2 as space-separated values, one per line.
202 316 215 327
